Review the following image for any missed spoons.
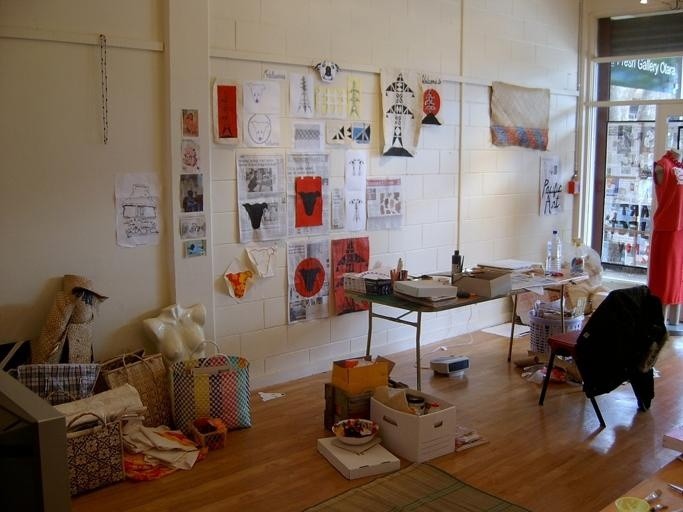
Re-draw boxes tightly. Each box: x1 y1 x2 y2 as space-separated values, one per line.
644 486 661 501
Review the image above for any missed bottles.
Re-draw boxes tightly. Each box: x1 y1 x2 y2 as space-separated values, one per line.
547 228 563 273
575 239 583 273
450 249 463 275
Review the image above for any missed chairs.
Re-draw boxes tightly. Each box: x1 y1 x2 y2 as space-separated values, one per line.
538 287 668 428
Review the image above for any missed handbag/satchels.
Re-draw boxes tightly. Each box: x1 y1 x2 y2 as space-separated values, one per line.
7 340 251 495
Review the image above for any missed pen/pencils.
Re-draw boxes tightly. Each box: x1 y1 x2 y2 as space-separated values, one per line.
390 269 408 284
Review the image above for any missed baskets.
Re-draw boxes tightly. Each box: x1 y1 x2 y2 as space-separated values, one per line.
528 310 584 360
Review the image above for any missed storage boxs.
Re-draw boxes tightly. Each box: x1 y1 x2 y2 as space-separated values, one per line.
332 355 396 397
371 389 456 463
324 380 407 433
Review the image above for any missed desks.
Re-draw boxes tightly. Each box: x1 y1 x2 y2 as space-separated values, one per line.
600 456 682 512
342 272 590 391
0 364 71 512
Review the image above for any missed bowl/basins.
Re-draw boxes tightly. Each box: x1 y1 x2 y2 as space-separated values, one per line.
613 497 650 512
332 417 379 446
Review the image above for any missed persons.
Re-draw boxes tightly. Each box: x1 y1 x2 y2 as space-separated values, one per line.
141 303 207 364
648 150 682 326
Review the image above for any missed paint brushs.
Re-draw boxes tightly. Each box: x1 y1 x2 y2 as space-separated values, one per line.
644 489 668 512
668 483 683 494
